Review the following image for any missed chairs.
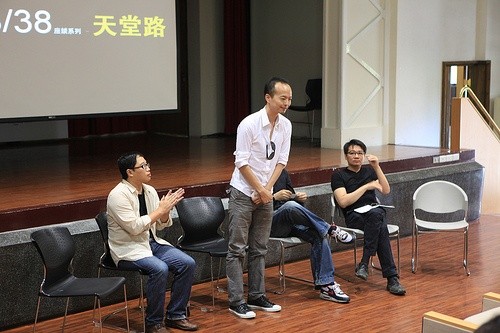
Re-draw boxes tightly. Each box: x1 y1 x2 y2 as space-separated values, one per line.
95 212 165 333
422 291 500 333
286 79 322 143
265 237 329 295
174 196 247 313
411 180 470 276
30 227 129 333
330 193 401 283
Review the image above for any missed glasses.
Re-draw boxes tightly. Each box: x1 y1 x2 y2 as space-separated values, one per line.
348 150 365 155
266 141 276 160
130 163 150 169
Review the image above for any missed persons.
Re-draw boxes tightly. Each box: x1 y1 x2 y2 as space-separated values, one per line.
270 169 356 302
226 76 293 318
330 139 406 295
106 152 198 333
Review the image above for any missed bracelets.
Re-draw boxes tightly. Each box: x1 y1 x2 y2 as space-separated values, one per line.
273 194 276 201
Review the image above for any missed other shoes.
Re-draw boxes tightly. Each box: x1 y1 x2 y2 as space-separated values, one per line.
355 263 369 281
145 321 174 333
164 318 198 330
386 277 407 294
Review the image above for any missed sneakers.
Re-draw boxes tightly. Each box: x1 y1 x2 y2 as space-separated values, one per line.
229 303 256 318
247 295 281 312
319 281 350 303
330 225 355 245
